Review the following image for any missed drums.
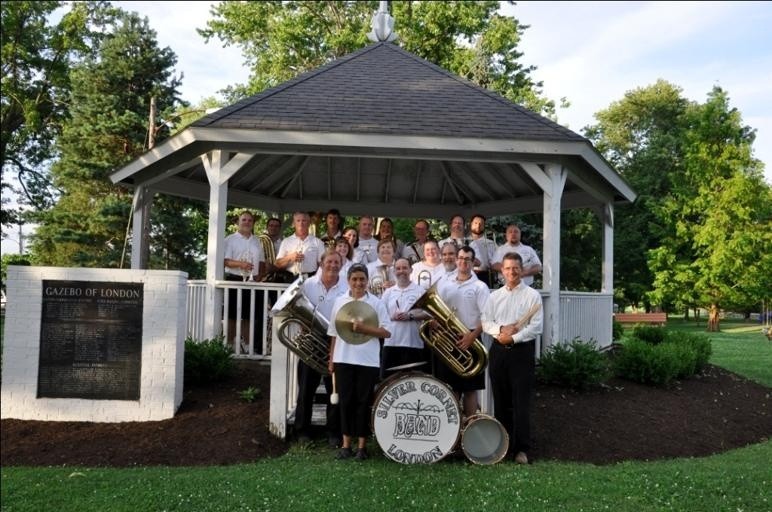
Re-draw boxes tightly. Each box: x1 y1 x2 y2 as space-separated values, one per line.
460 413 509 465
370 370 463 464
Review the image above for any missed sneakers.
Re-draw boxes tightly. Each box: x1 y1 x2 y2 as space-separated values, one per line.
516 452 528 464
354 449 366 461
335 447 351 459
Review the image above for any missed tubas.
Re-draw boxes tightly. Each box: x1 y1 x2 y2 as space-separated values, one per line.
269 275 331 377
408 276 490 377
230 215 277 283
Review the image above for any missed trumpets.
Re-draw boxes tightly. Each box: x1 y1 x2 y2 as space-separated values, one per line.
239 246 255 281
370 263 388 297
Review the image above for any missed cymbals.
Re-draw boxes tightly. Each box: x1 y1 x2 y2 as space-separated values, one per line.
335 300 378 344
385 361 426 370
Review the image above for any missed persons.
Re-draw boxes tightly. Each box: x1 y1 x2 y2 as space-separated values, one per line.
439 246 489 426
381 257 428 382
222 211 265 355
328 264 392 463
257 218 283 358
480 253 543 465
274 209 541 285
300 252 347 440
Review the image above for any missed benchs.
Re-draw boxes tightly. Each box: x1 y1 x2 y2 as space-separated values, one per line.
613 312 668 329
